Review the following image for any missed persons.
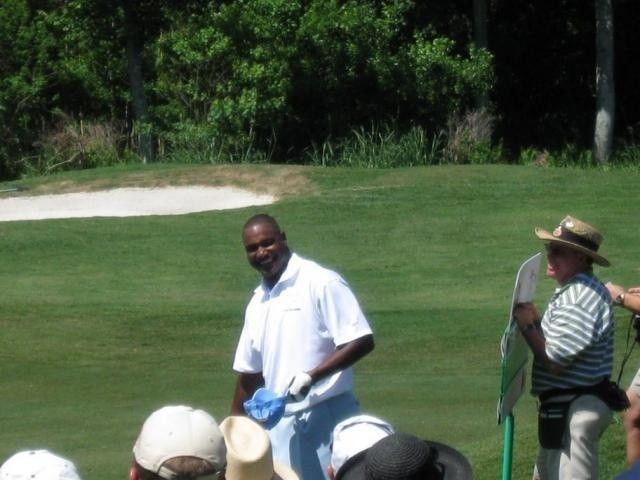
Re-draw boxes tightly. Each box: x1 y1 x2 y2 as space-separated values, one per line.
513 215 614 480
335 431 473 479
605 281 640 468
328 414 396 480
232 213 375 480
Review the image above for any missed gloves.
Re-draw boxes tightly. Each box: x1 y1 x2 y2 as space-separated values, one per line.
285 374 314 403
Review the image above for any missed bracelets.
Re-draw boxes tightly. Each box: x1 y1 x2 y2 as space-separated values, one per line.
534 319 540 329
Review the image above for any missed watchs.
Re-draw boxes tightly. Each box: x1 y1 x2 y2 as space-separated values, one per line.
522 323 536 333
616 291 625 306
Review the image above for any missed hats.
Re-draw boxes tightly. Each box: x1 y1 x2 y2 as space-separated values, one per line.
535 214 610 267
329 415 474 480
243 387 290 430
